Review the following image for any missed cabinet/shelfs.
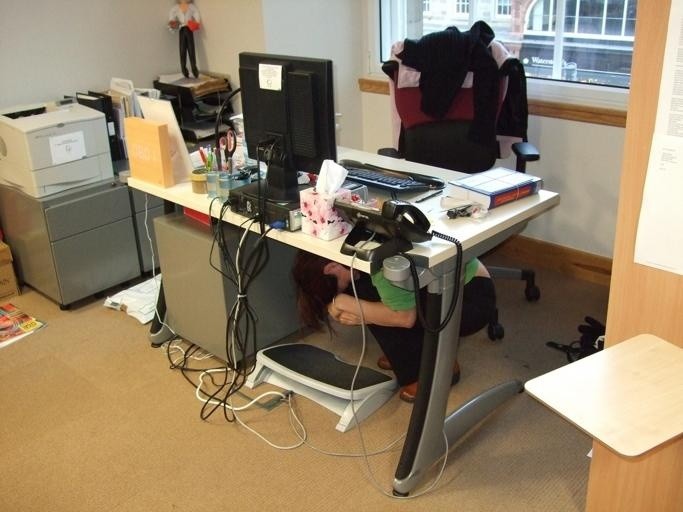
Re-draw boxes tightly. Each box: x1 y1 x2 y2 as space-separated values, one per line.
2 181 161 308
153 77 235 145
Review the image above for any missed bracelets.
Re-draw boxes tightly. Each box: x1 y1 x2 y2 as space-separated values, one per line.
331 293 337 310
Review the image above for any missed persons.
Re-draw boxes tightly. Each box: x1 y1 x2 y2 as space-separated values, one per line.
298 248 497 402
160 0 206 81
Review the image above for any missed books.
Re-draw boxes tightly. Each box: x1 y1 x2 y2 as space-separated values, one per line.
439 191 471 210
2 303 47 353
446 166 546 203
78 83 190 190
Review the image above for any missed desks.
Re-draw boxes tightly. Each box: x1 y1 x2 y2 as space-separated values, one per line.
123 125 564 497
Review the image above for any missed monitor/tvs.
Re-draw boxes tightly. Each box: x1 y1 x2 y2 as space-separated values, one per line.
238 50 337 204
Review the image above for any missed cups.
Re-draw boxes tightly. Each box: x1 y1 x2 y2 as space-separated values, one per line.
191 168 209 194
208 171 253 205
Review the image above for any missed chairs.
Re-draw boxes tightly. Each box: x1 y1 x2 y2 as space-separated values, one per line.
373 57 540 343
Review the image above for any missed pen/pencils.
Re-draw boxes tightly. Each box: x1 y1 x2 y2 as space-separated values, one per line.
415 190 443 203
199 149 207 171
207 143 213 172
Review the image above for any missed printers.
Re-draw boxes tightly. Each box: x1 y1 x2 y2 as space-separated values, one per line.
0 101 113 199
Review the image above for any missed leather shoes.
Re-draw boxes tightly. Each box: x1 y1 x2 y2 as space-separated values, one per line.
399 361 460 404
377 354 394 371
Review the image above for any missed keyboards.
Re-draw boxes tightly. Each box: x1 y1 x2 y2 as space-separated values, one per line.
338 159 446 192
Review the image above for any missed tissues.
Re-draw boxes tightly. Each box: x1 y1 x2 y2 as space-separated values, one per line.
299 158 351 241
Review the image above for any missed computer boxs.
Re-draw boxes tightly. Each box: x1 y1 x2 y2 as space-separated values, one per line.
228 179 314 233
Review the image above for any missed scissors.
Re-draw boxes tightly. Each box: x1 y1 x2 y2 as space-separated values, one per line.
216 128 237 188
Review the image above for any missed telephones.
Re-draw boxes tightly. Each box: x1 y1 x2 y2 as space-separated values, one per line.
333 198 434 262
